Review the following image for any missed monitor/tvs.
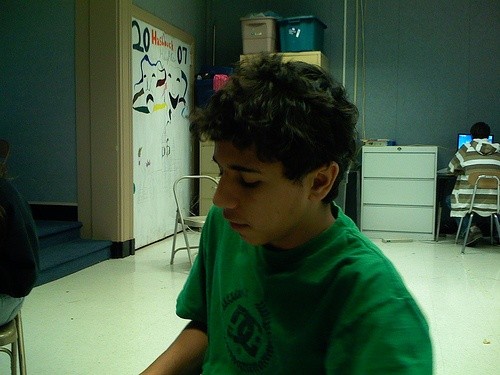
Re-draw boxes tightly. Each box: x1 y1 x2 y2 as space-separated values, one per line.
456 133 494 153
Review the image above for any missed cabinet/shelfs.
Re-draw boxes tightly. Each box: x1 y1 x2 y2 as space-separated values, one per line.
199 133 221 215
360 145 438 242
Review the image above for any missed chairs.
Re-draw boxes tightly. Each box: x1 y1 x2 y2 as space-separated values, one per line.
455 175 500 254
170 176 220 266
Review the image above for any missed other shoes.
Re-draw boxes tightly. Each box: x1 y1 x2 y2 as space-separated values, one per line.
462 226 482 246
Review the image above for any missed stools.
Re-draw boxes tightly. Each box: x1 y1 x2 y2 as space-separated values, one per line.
0 312 27 375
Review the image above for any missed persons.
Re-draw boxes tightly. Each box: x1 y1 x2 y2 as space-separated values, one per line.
448 121 500 246
0 141 40 326
139 56 434 375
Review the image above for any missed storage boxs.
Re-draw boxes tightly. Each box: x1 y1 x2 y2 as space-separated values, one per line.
195 67 235 106
240 15 328 75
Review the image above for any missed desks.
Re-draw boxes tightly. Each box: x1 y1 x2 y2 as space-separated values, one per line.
436 168 457 241
